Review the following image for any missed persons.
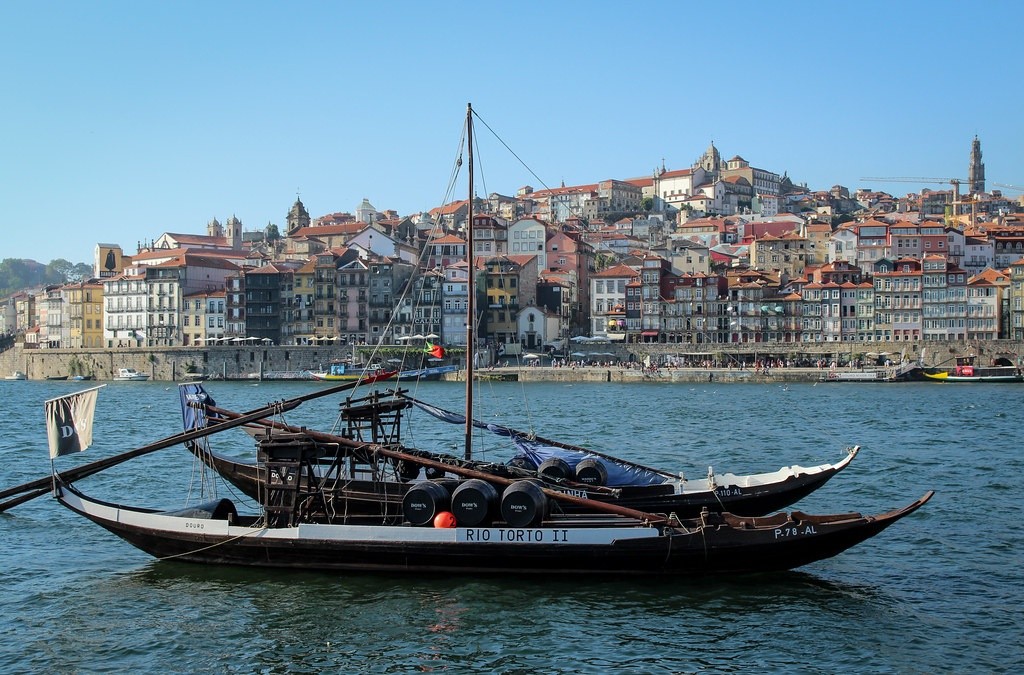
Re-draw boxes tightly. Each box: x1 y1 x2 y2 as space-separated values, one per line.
497 359 509 368
551 358 890 370
348 339 370 346
528 359 540 369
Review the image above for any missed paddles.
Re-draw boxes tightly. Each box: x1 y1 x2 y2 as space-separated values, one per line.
0 368 399 515
0 398 303 499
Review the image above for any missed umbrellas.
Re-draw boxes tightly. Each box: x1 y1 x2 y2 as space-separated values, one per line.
398 333 439 346
308 336 345 345
523 352 538 365
866 351 893 365
570 336 615 366
178 382 222 430
192 333 272 346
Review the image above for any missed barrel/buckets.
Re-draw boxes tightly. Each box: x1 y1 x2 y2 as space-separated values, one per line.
537 457 574 481
501 480 548 527
574 458 608 487
505 454 538 470
451 478 499 527
402 481 450 525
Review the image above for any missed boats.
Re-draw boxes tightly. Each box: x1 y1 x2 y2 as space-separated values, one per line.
113 368 150 381
71 375 91 381
306 335 425 382
5 371 28 380
917 339 1023 383
45 376 69 380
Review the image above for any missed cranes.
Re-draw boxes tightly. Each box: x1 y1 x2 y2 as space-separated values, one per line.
861 174 974 184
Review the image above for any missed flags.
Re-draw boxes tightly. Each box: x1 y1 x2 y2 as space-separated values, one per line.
45 388 99 460
423 341 445 358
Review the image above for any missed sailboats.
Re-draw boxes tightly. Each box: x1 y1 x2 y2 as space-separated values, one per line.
43 101 937 587
